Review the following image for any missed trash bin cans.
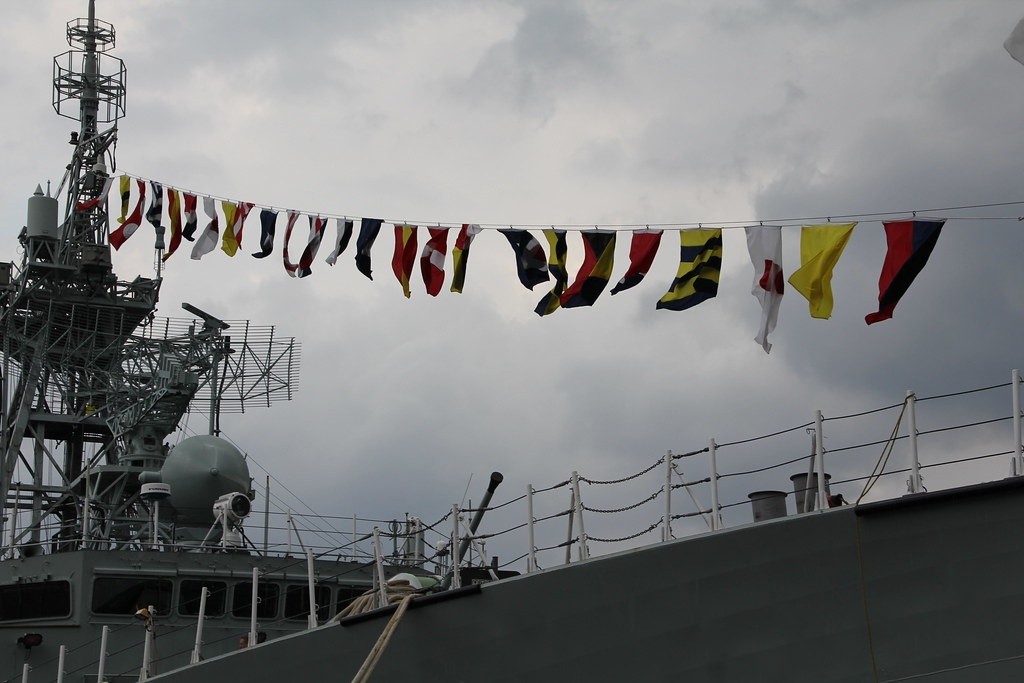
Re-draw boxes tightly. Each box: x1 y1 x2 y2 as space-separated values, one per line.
748 489 788 522
790 470 833 514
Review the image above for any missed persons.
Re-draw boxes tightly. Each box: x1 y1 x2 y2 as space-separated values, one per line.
239 636 249 650
257 631 268 645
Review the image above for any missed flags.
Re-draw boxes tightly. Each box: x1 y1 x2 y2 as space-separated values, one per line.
610 229 664 295
864 215 948 326
82 171 615 317
655 228 722 311
787 220 858 319
744 225 783 353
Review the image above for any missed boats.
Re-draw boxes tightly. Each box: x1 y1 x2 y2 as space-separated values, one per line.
0 0 1024 683
